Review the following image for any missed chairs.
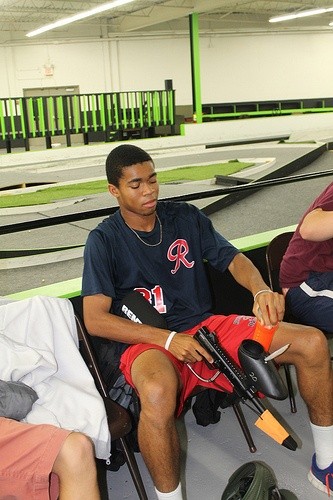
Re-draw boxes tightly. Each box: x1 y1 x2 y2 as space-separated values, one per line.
0 230 297 500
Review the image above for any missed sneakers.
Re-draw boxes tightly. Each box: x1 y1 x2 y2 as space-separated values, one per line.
308 453 333 498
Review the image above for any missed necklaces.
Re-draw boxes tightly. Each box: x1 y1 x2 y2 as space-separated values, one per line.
125 212 162 246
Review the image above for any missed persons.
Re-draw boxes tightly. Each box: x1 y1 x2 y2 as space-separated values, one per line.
0 380 101 500
280 182 333 339
81 144 333 500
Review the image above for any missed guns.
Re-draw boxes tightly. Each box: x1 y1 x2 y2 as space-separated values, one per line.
191 326 301 454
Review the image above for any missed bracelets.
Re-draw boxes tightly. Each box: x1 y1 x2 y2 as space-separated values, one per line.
165 331 177 351
254 290 273 301
